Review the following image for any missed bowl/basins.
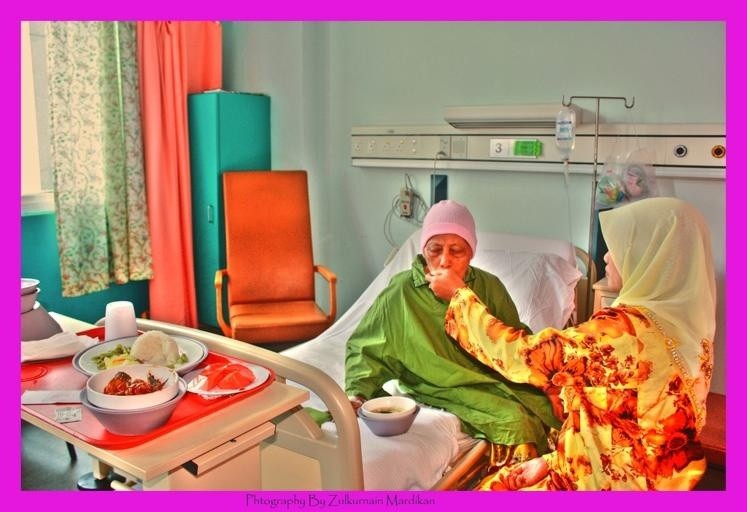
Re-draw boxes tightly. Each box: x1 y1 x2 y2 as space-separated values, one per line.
79 375 187 434
361 396 416 418
20 288 40 314
357 405 419 436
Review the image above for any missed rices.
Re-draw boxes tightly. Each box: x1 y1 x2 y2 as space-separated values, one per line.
129 329 179 367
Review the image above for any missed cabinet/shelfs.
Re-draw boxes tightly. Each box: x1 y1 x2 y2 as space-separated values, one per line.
179 81 276 332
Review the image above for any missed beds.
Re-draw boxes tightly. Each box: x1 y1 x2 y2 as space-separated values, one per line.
93 213 604 492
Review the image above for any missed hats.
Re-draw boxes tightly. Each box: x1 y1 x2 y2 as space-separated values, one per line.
421 198 477 258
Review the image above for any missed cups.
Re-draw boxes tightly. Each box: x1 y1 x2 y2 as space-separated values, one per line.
104 301 138 342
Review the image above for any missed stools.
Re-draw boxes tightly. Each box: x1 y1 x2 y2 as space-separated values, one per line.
697 391 725 471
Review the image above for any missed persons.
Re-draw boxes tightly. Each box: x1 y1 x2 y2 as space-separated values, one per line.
345 201 565 458
426 197 718 490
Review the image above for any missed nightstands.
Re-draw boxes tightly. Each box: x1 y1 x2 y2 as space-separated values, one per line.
590 274 621 315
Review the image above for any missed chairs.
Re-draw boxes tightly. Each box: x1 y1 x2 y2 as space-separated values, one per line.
207 163 338 353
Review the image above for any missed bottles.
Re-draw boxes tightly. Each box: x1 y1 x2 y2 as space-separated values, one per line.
555 107 576 158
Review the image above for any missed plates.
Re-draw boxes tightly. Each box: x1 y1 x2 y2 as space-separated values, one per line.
70 334 208 378
85 363 178 410
20 277 40 295
183 363 269 394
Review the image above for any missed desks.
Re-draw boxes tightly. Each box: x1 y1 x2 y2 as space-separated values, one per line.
20 306 312 494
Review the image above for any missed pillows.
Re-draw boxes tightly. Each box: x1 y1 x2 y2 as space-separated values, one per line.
456 240 584 343
